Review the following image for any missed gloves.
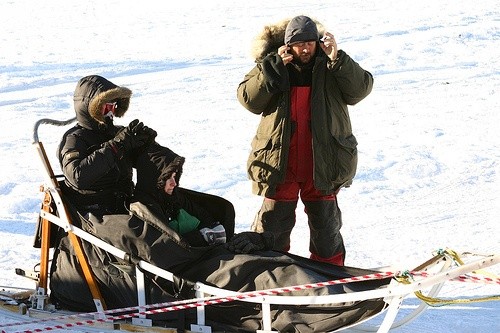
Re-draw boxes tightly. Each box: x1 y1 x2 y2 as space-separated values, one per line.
112 119 157 160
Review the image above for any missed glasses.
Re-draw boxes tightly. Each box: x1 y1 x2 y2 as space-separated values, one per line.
101 102 118 116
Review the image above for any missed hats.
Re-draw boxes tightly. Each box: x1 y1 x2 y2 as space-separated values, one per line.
284 15 320 47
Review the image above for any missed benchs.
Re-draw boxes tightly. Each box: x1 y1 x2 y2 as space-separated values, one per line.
42 185 236 250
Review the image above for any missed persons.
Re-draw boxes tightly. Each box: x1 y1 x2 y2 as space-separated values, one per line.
235 16 373 267
58 75 158 267
127 144 227 286
